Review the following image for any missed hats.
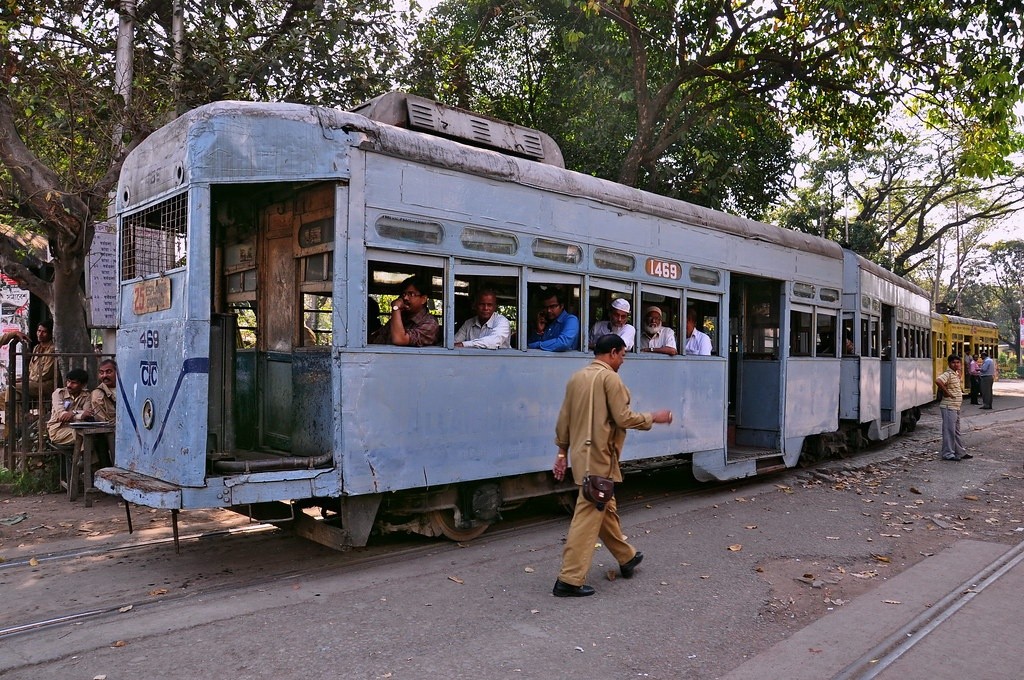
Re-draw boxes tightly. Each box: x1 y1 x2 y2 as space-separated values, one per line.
611 298 631 313
646 306 662 316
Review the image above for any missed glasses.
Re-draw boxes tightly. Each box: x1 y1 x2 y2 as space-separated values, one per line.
544 302 562 311
401 291 422 299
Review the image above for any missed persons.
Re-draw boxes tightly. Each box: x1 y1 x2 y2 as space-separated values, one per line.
958 348 973 388
552 334 673 597
818 329 923 358
0 320 116 472
968 353 982 405
975 352 994 410
935 354 973 461
368 275 713 357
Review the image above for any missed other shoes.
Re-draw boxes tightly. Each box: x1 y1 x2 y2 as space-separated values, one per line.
970 402 982 405
943 456 961 460
979 404 992 409
90 464 99 474
77 455 84 468
961 454 973 459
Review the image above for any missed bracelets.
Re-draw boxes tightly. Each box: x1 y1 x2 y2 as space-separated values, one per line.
651 348 654 352
557 454 567 458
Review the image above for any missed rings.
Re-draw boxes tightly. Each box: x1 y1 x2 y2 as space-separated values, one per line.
670 412 671 415
669 415 671 419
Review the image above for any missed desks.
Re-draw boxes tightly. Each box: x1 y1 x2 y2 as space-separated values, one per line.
71 425 114 506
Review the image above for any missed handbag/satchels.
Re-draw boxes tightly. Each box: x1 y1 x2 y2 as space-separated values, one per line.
582 475 614 504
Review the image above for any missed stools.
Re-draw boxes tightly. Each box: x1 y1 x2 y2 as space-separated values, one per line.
51 442 72 495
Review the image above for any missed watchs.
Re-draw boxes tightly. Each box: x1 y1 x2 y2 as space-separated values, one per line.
392 306 403 311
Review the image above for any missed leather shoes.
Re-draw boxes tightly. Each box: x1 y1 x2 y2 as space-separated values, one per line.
620 552 643 578
553 579 595 597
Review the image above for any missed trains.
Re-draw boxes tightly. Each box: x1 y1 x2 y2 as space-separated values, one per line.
92 92 934 546
930 310 1000 394
